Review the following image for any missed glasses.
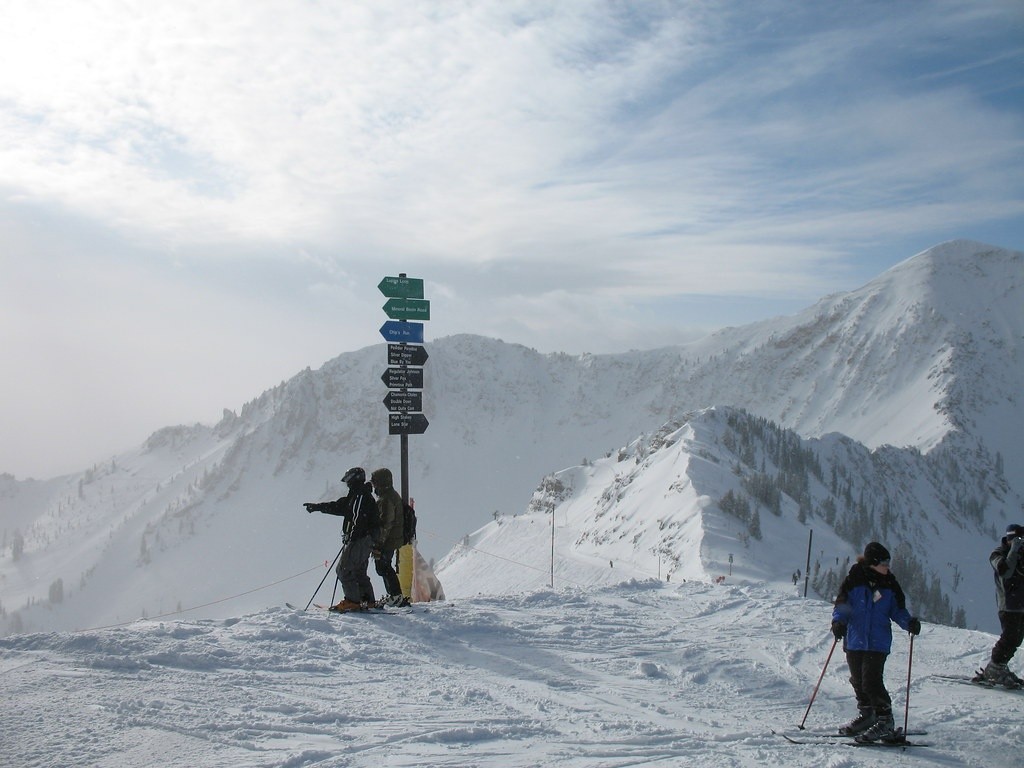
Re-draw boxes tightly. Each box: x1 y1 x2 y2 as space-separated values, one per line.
1015 527 1024 536
876 559 890 566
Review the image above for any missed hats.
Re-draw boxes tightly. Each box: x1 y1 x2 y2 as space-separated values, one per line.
1006 523 1022 541
864 542 890 564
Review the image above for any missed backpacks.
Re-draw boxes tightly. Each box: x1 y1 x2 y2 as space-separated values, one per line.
399 497 417 546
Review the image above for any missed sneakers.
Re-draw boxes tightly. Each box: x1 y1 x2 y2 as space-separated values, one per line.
361 600 376 610
378 595 405 607
845 714 875 733
336 599 361 610
984 661 1018 685
863 715 894 739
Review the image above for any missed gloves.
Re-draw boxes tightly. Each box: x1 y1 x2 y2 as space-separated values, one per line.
372 545 382 560
303 503 319 512
832 622 845 639
908 618 922 635
342 533 352 544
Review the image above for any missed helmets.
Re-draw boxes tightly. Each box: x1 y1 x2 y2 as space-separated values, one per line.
341 467 365 487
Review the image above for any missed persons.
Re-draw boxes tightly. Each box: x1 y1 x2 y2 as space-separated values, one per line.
303 467 377 613
370 468 404 606
831 541 921 744
982 524 1024 690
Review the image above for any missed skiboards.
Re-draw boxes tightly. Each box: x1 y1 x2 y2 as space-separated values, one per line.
771 727 929 747
932 672 1024 690
285 603 429 614
